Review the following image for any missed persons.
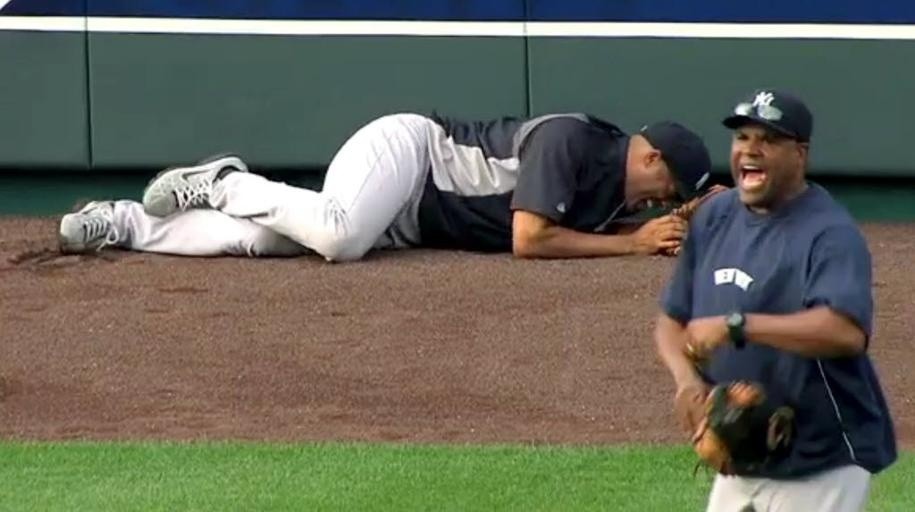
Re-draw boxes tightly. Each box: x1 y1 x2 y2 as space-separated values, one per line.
56 107 731 260
652 87 901 511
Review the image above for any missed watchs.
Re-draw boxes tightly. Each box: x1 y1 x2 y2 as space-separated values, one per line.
724 310 747 349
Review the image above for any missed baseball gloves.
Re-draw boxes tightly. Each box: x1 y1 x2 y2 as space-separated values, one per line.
685 379 797 478
656 183 733 256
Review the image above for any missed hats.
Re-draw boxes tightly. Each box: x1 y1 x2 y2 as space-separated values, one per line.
722 90 813 143
641 119 713 202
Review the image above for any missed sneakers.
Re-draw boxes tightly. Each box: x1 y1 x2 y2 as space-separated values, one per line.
55 200 115 257
141 152 249 216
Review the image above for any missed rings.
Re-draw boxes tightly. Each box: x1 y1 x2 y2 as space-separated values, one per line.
686 344 694 354
